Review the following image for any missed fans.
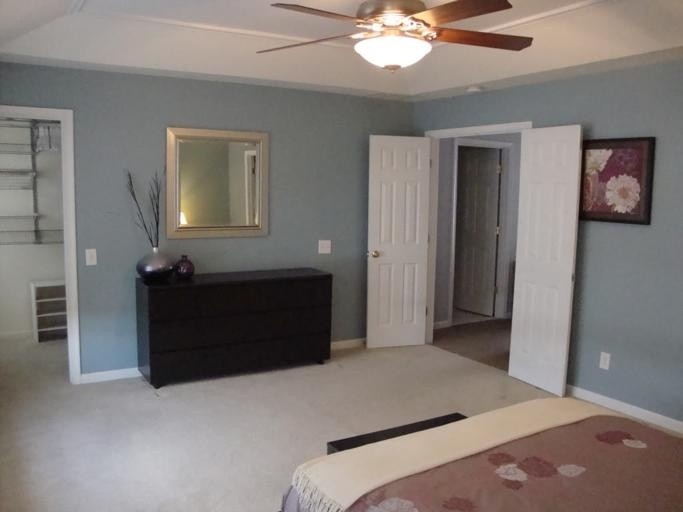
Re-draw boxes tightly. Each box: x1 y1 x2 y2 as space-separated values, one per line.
255 0 533 110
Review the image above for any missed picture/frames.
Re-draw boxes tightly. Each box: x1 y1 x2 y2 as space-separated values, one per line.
580 136 655 226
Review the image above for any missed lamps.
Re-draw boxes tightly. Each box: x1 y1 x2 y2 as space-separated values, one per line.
352 0 435 74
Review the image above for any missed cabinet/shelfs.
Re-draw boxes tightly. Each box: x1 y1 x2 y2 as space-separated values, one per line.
31 280 66 343
134 268 333 389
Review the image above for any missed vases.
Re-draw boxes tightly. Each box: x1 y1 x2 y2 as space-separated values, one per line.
174 254 195 282
137 246 175 286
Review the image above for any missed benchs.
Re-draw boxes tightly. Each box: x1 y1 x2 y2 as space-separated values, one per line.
328 411 469 468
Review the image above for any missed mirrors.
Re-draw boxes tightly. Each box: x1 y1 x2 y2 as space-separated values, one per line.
166 126 269 240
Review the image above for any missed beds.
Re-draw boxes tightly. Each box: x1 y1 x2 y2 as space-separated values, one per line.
286 397 681 510
